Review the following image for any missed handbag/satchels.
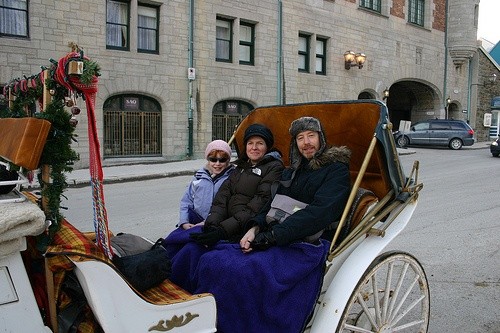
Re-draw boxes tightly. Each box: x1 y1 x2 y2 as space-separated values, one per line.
112 237 172 292
267 193 325 242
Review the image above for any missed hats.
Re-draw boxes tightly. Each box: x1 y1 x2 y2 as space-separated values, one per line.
288 117 326 170
242 123 275 148
205 140 232 161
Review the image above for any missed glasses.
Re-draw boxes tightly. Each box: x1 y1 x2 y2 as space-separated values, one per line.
207 157 230 163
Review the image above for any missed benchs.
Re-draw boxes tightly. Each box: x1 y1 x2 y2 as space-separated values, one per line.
0 117 52 252
18 190 217 333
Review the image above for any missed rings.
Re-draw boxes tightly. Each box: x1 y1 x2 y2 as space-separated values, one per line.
248 241 251 243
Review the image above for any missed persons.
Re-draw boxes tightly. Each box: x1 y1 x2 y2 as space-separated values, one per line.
239 117 350 254
189 124 286 246
179 140 235 231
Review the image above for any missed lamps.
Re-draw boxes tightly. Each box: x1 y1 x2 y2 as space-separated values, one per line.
344 50 367 70
382 87 390 100
446 96 451 107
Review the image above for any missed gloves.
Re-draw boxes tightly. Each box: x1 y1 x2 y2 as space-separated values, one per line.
249 231 274 252
188 225 223 252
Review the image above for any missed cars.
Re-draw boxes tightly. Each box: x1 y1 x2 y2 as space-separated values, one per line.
393 119 475 150
